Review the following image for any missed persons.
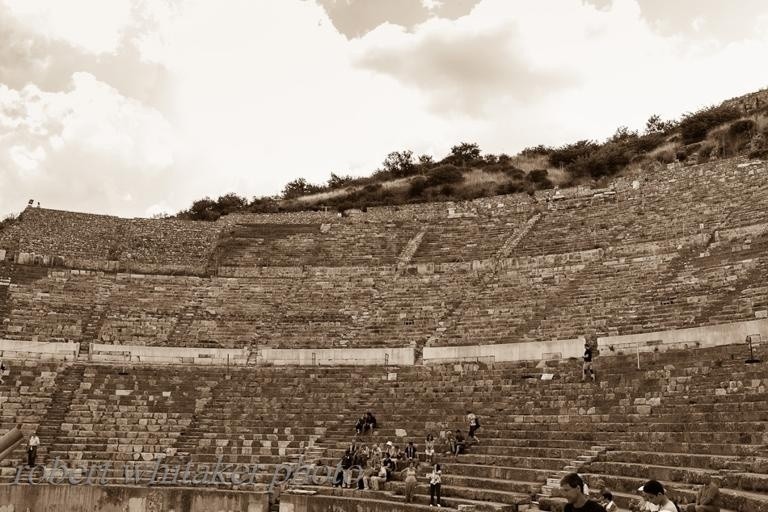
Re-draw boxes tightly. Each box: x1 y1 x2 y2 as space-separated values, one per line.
466 409 480 444
27 430 40 467
559 472 608 511
582 476 679 512
581 343 595 383
332 411 467 507
